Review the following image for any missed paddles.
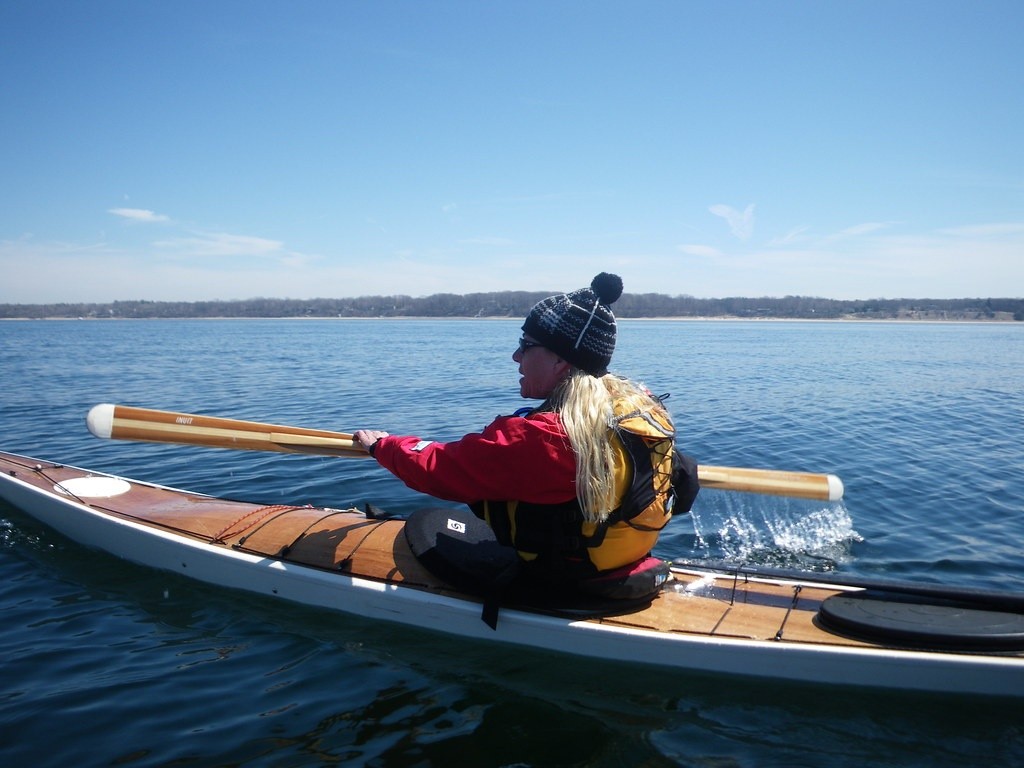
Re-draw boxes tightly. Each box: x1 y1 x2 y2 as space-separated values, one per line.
85 401 844 503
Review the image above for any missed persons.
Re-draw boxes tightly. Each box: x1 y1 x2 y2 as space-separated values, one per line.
352 287 702 612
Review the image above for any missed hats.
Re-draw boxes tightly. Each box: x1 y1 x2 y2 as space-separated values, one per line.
521 272 624 371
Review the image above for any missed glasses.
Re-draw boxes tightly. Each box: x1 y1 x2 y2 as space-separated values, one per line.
518 337 546 354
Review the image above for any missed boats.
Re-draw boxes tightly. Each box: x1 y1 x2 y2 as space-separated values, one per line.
0 450 1024 699
